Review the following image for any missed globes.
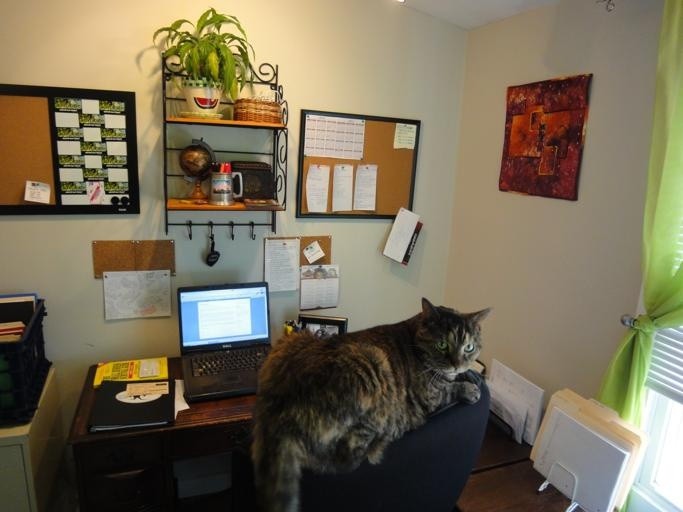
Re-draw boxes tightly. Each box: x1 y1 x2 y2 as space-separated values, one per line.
179 137 215 199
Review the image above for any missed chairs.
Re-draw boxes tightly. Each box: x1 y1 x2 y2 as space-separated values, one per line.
297 381 491 512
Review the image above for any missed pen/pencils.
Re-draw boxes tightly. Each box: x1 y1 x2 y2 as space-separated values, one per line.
283 319 303 335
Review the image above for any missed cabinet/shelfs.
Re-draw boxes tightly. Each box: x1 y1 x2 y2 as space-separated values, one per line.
159 50 290 242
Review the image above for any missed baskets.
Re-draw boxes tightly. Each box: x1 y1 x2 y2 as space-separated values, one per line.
0 299 52 425
233 98 282 124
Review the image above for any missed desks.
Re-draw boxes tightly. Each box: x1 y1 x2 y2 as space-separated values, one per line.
66 356 259 512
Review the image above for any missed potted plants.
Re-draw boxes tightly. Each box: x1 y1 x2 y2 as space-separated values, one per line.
153 6 256 117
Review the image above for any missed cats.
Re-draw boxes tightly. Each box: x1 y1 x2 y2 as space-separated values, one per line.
250 296 495 512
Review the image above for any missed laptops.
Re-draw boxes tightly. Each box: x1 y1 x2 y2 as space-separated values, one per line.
177 283 275 402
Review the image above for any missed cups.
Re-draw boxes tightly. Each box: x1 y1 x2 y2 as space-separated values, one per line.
210 170 243 205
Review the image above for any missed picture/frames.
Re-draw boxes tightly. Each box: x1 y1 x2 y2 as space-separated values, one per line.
297 313 348 337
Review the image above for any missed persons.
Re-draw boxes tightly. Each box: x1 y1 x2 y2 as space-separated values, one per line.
314 324 329 339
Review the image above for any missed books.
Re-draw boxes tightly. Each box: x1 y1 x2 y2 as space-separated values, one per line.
0 293 37 345
87 378 175 432
